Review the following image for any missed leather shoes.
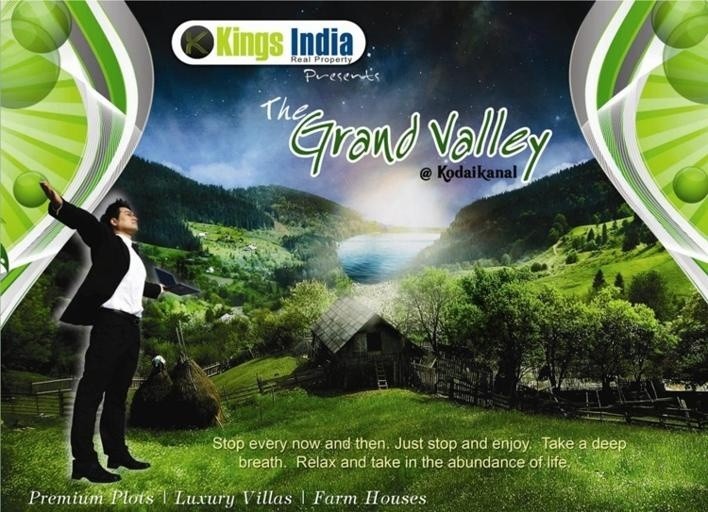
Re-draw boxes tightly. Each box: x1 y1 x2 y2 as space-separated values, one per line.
71 457 122 483
108 450 150 469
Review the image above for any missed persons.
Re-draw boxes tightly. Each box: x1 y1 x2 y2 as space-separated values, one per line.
38 179 173 487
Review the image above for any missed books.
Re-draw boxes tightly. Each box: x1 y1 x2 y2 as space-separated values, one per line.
153 265 201 297
